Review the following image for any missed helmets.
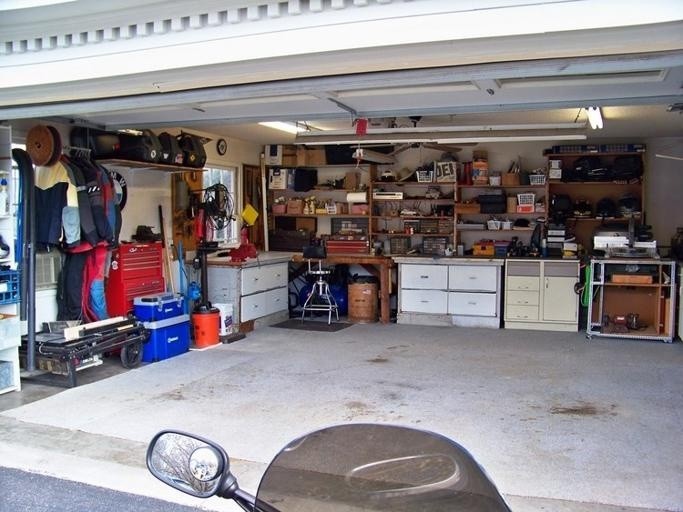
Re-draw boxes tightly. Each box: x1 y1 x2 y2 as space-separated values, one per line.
574 193 641 219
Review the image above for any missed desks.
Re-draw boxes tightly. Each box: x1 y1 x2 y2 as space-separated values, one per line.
274 252 394 324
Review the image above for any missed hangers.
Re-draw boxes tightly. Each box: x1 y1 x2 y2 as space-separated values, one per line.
58 143 91 161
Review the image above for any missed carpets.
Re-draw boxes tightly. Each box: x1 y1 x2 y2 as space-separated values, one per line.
2 318 683 512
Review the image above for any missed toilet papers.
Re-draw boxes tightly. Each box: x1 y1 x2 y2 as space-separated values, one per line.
346 191 368 203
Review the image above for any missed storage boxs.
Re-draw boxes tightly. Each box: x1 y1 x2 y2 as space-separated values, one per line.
131 292 191 362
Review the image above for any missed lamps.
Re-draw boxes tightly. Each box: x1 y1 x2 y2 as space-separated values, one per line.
584 105 604 131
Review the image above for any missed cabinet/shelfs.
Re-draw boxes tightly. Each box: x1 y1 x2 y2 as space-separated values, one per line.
189 255 290 328
0 126 22 394
391 256 676 343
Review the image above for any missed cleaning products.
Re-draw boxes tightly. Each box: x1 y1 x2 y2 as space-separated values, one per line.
303 195 316 215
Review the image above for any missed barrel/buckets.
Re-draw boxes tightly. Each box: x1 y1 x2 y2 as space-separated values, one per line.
209 303 235 338
192 301 220 347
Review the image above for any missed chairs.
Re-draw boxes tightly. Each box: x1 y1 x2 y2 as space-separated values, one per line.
301 245 341 324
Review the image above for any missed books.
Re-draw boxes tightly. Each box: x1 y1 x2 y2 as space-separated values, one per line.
389 219 453 256
